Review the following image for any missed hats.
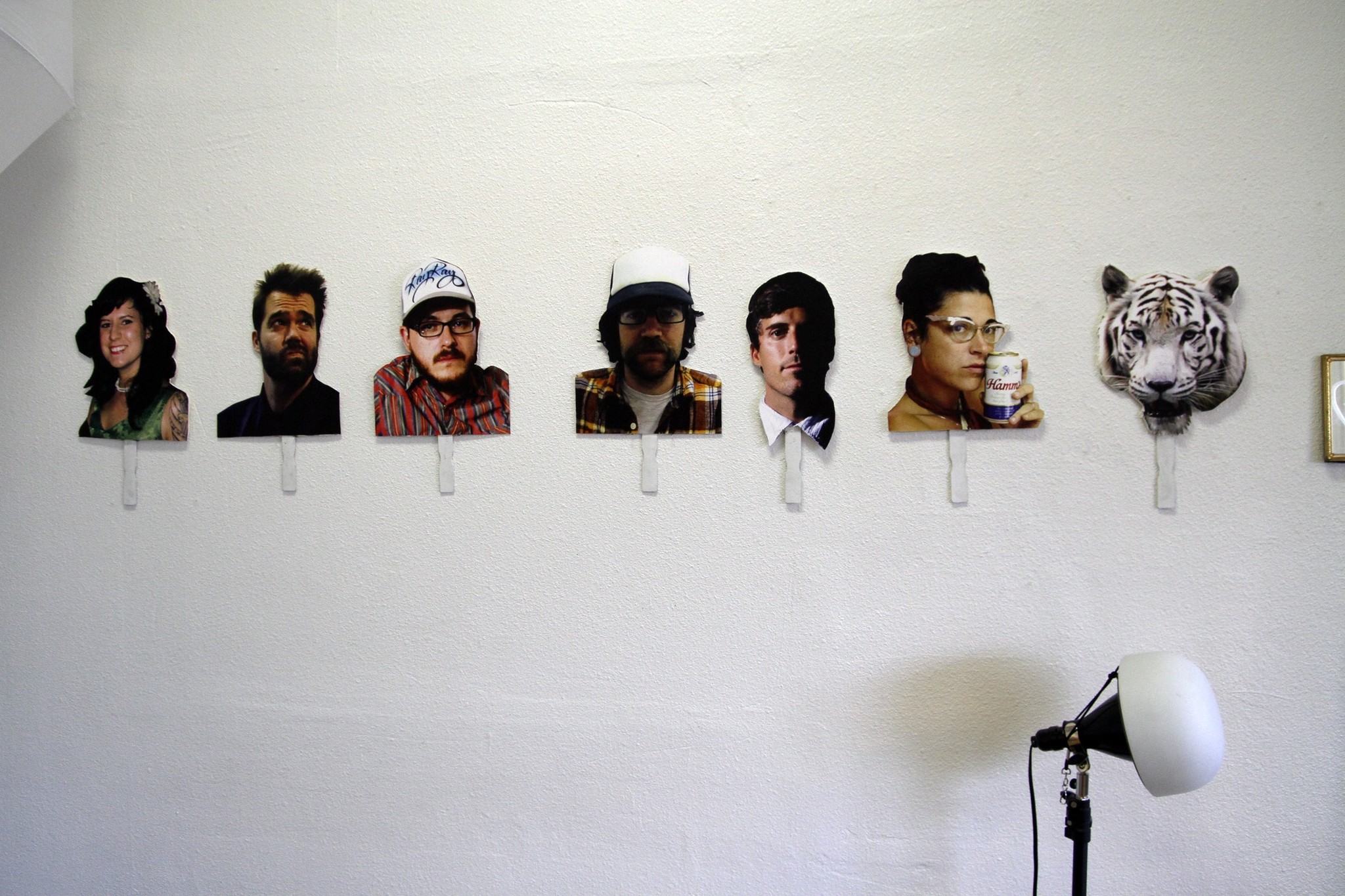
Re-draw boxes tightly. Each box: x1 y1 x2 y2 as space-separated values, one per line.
400 256 475 323
606 245 694 308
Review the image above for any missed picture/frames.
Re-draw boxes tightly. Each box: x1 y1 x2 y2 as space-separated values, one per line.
1320 354 1345 463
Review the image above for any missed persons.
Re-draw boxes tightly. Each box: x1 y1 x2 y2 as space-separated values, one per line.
888 252 1046 431
745 271 837 454
373 258 511 436
76 278 192 444
576 252 723 437
218 265 343 440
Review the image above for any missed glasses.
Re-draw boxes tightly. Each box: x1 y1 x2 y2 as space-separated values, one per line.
617 304 689 325
926 317 1009 346
403 314 478 338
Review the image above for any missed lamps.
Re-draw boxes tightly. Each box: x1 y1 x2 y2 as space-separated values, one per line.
1029 652 1227 896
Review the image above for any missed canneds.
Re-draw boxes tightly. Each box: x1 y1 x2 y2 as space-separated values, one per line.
984 352 1022 423
